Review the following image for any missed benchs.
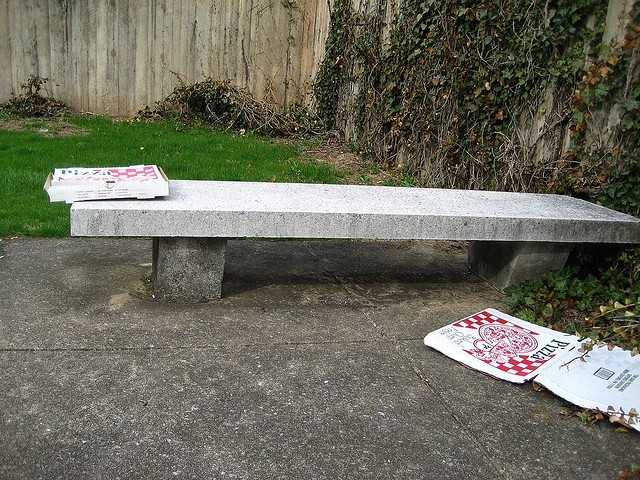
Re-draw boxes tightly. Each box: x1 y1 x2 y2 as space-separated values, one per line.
71 179 639 300
